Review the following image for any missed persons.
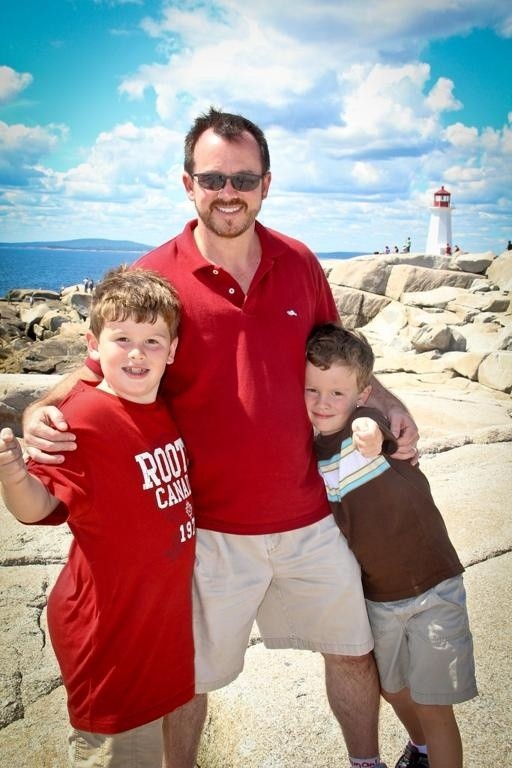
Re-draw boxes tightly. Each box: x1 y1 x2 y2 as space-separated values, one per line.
28 287 42 307
0 261 196 768
21 107 422 768
7 289 16 305
444 243 452 255
454 244 461 252
506 239 512 251
74 276 94 293
385 236 411 254
303 323 477 768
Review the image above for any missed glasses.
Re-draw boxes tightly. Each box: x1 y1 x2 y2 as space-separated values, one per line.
194 173 266 192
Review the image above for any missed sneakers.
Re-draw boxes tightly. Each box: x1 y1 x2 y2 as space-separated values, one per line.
394 740 429 768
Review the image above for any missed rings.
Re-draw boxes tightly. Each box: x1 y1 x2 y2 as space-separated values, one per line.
412 447 418 454
